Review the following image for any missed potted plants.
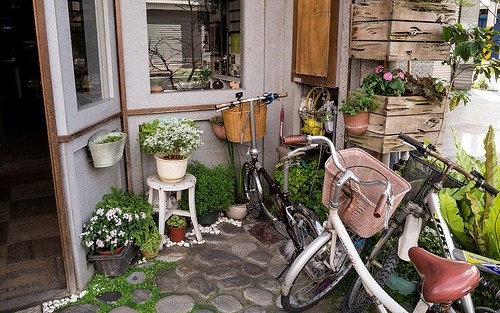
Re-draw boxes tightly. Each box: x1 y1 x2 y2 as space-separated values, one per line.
220 141 248 220
178 160 236 225
132 230 162 259
211 115 229 140
88 129 128 168
151 76 163 93
340 82 380 136
166 215 187 242
385 229 444 296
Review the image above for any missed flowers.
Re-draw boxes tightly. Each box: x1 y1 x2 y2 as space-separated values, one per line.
78 208 147 254
138 117 204 160
361 65 448 108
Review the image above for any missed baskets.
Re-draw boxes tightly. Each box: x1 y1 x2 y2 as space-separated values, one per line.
322 147 412 238
223 100 266 142
299 109 327 137
403 153 463 206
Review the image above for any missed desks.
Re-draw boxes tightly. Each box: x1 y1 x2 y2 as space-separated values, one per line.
210 75 241 89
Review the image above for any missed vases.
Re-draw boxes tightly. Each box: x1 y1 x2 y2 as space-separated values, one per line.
345 91 449 154
349 0 456 61
96 245 125 255
87 240 135 277
153 152 190 183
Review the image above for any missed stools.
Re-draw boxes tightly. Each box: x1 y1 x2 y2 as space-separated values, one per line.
147 173 203 241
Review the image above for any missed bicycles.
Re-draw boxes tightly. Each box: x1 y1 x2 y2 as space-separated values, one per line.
214 91 335 285
346 132 500 313
280 133 480 313
